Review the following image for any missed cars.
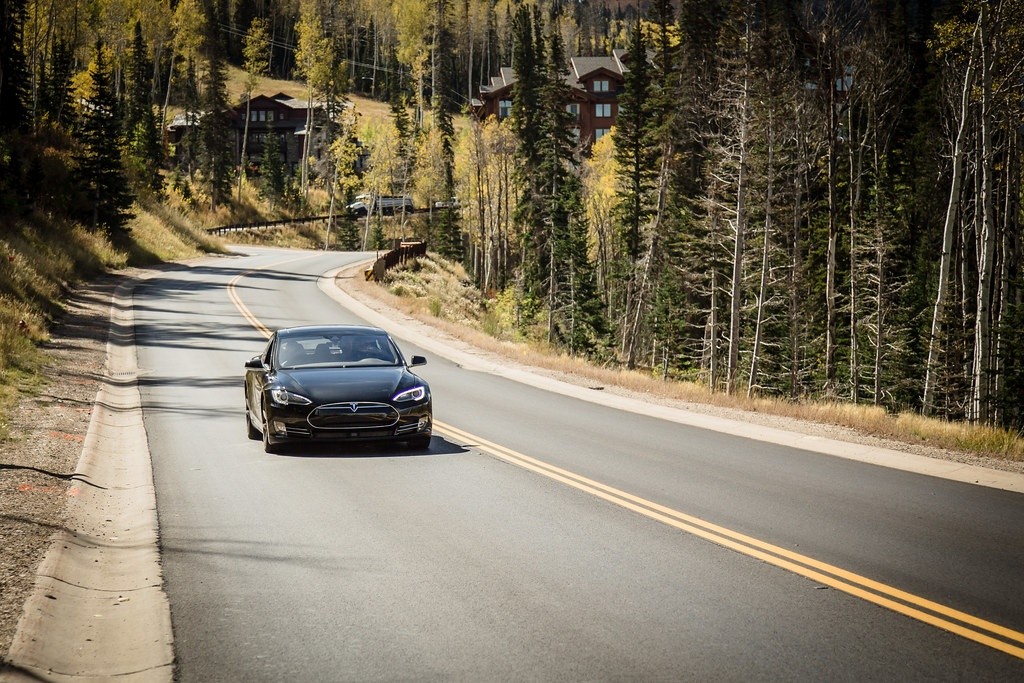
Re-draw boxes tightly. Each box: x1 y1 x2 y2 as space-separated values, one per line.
435 196 467 211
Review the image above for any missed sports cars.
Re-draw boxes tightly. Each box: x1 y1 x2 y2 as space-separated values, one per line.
243 324 432 455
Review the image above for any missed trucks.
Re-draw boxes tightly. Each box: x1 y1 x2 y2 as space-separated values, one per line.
346 194 414 216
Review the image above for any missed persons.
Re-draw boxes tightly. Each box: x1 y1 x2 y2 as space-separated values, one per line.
356 338 373 354
282 341 299 366
